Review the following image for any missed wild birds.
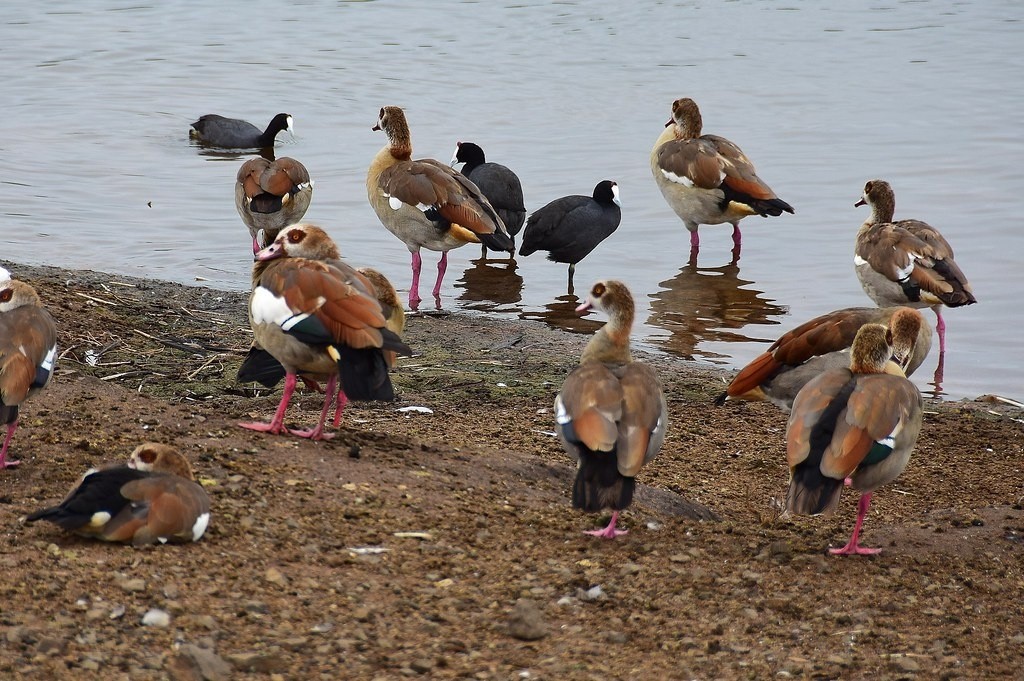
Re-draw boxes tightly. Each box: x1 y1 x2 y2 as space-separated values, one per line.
712 304 931 553
553 279 668 537
519 180 624 279
27 442 210 545
449 141 528 254
367 106 515 300
0 280 57 466
853 179 978 353
649 98 794 244
188 113 412 439
